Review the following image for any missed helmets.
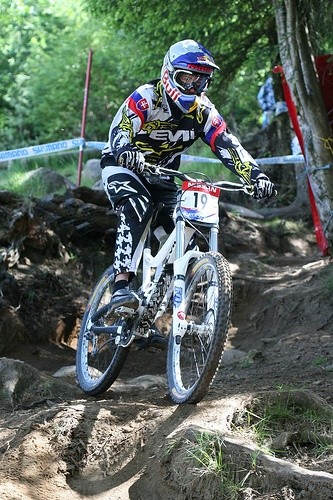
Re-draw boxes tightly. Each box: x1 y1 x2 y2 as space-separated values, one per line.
160 40 220 114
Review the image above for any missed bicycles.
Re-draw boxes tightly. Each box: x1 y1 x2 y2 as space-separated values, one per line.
74 162 277 405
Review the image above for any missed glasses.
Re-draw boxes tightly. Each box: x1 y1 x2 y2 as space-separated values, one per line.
173 69 209 93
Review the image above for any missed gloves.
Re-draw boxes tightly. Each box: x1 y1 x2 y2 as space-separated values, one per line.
117 151 145 174
251 177 275 200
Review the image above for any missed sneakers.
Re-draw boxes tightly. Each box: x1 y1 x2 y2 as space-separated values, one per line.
106 288 139 319
138 328 168 353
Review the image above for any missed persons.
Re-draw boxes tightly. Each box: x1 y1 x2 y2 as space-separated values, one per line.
100 38 279 353
268 52 295 154
255 77 284 156
